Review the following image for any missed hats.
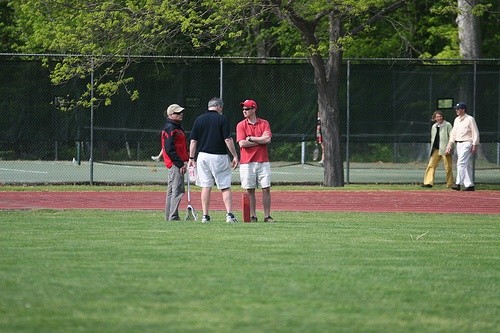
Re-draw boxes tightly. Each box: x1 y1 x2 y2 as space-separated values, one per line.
240 100 257 110
453 102 466 109
166 104 185 114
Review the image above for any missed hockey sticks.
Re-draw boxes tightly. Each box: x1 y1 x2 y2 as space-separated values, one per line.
184 151 198 221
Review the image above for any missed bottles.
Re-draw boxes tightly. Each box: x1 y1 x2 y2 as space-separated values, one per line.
189 165 196 182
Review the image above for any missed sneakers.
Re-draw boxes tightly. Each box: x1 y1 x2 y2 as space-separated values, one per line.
226 213 238 223
250 216 257 222
202 216 210 222
264 216 275 222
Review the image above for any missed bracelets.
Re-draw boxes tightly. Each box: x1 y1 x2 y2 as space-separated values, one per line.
188 156 194 160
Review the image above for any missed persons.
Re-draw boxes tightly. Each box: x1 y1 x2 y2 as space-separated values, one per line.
161 104 190 222
151 148 164 163
419 110 455 189
316 110 325 165
446 102 479 192
236 100 277 223
187 97 239 223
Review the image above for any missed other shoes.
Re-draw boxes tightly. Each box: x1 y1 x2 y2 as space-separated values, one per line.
463 186 475 191
319 161 324 166
452 185 460 190
421 184 432 188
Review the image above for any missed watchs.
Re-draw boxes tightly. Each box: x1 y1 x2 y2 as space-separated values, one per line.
247 136 251 142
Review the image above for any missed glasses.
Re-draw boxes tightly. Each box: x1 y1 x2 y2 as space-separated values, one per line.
173 111 184 115
241 108 254 111
